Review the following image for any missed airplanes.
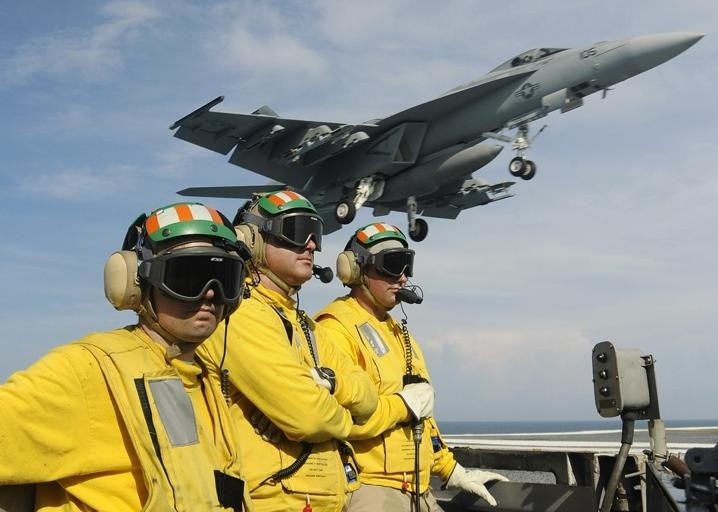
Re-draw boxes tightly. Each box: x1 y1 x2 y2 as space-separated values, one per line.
169 29 706 242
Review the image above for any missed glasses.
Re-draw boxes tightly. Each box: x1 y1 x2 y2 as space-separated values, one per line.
351 238 417 279
240 210 326 251
137 245 247 306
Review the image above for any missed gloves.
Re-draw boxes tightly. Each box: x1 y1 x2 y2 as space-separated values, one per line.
445 462 509 508
394 382 435 422
251 366 332 444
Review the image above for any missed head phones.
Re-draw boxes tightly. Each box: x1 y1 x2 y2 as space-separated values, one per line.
103 209 258 317
232 192 265 271
336 235 360 285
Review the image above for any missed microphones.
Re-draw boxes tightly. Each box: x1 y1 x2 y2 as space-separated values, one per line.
313 264 333 283
398 289 423 304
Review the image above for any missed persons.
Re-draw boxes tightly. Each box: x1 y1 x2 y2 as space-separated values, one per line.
312 222 509 512
0 202 261 512
198 191 381 511
